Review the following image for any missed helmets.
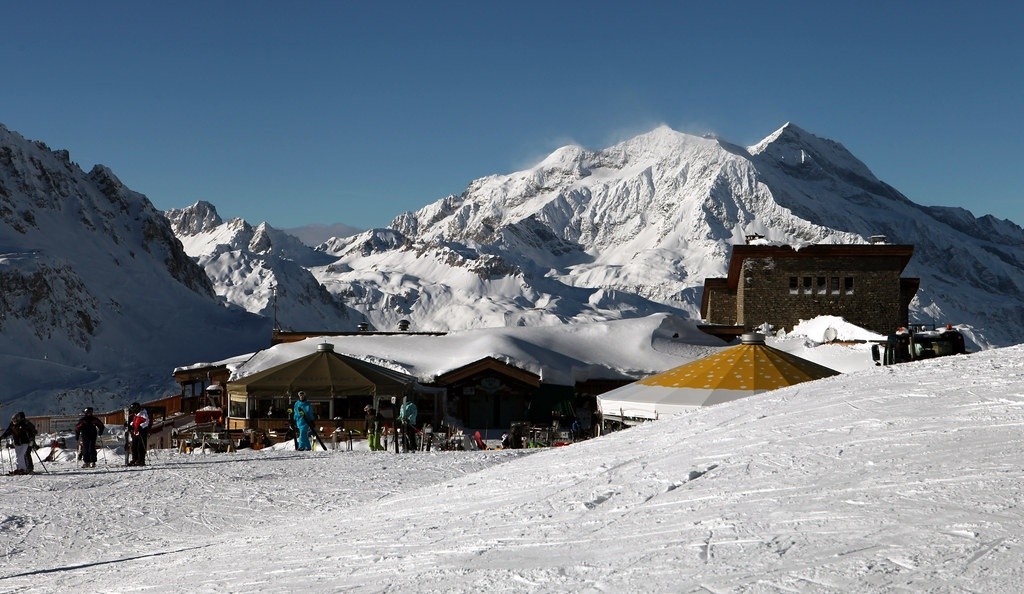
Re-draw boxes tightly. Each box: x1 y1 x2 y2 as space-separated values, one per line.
364 405 373 411
298 391 306 396
84 407 93 412
129 403 140 410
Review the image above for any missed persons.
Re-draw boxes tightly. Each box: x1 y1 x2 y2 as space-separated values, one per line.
124 402 152 467
572 419 580 443
395 394 418 453
74 406 105 468
0 412 38 471
363 404 384 451
294 390 315 450
9 413 29 474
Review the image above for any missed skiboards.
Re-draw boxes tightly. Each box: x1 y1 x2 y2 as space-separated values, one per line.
391 394 408 454
287 403 329 451
124 421 129 468
373 397 382 450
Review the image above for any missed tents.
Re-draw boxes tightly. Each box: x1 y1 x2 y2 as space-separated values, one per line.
226 343 416 434
596 331 841 441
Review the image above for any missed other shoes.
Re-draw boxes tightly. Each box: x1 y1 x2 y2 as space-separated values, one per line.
305 447 311 451
127 460 144 466
82 463 96 468
298 448 305 451
